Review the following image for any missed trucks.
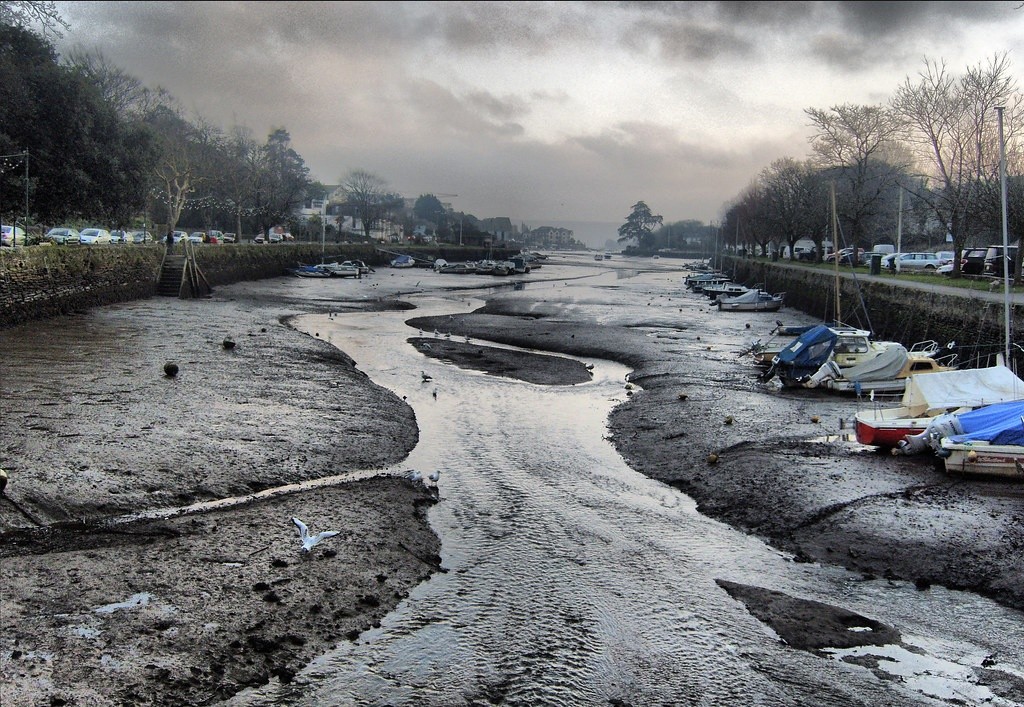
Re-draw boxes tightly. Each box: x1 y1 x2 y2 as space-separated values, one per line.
793 240 834 261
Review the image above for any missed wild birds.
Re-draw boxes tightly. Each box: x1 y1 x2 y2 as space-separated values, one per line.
465 335 471 341
432 388 439 397
444 332 451 338
434 329 440 336
292 517 340 551
419 328 423 334
421 370 433 381
428 470 443 487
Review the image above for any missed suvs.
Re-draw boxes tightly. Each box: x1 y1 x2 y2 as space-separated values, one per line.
827 247 865 262
982 245 1018 277
960 247 988 274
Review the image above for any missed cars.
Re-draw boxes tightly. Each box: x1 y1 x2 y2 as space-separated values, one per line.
188 230 295 244
735 245 956 274
163 231 188 244
80 228 113 245
133 231 154 244
46 228 81 246
110 230 133 244
0 225 26 247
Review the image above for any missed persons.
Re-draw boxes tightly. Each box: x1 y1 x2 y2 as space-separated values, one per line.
167 231 174 255
202 233 218 243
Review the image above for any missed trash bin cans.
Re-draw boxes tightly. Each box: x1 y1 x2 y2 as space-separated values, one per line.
738 250 747 256
772 251 778 260
870 255 881 274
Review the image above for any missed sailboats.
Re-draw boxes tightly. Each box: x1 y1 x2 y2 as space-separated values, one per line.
509 225 547 269
852 106 1024 447
738 177 878 335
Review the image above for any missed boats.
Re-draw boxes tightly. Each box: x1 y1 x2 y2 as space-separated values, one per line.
289 259 375 278
890 400 1024 482
390 255 528 276
754 325 959 395
594 251 660 260
680 261 788 312
